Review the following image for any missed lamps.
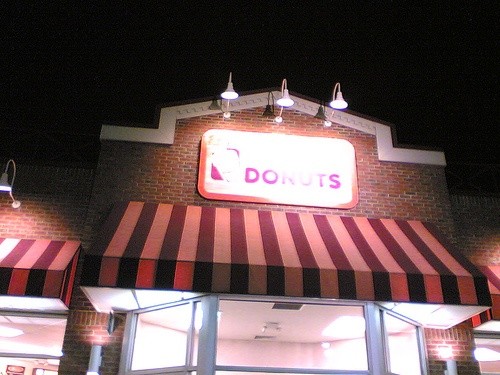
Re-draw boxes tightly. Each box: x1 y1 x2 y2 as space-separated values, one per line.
0 159 22 209
274 79 294 123
219 72 239 118
324 82 349 128
